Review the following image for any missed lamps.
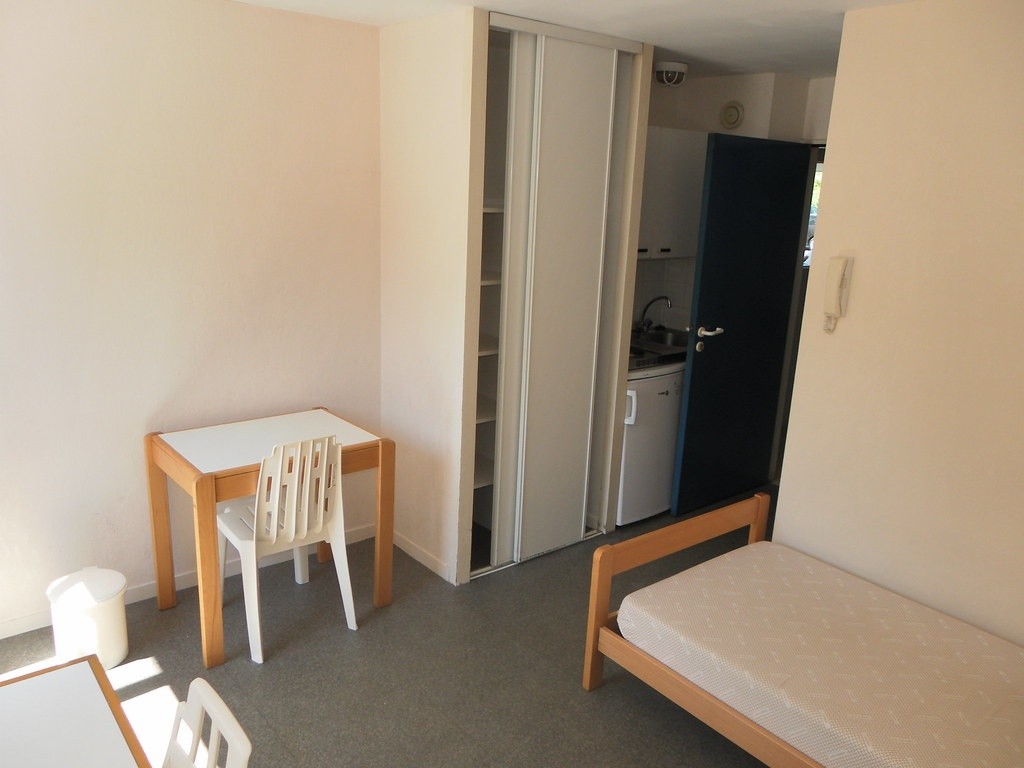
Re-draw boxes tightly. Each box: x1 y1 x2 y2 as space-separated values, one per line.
654 62 688 89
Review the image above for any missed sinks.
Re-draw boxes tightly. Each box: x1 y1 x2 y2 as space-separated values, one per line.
644 331 688 348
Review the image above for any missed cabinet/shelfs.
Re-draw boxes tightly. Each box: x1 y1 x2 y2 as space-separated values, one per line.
638 126 708 259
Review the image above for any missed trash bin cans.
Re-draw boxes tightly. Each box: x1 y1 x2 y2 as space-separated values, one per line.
47 566 129 671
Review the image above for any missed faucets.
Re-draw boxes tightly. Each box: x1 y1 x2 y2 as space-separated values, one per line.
642 295 672 321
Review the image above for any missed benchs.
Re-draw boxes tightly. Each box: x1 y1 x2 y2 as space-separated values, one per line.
581 493 1023 768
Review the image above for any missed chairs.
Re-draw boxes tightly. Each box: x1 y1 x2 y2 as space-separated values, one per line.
163 677 253 768
376 5 653 587
216 434 359 664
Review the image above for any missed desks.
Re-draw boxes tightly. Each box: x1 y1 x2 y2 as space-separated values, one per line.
0 654 152 768
143 406 395 670
